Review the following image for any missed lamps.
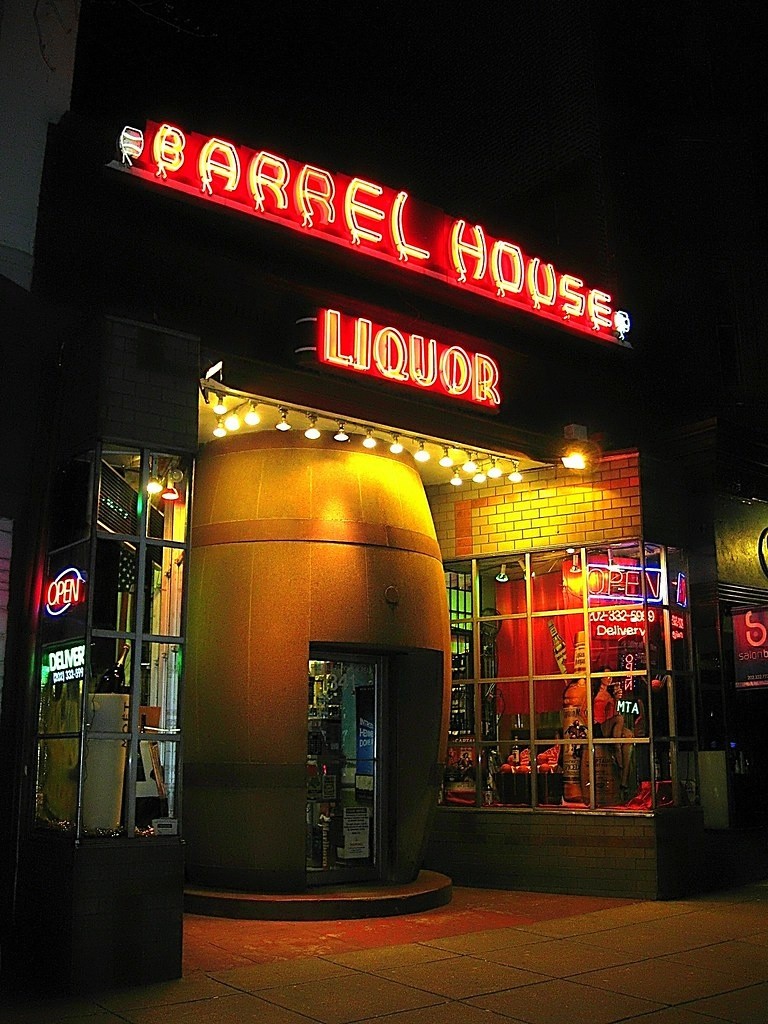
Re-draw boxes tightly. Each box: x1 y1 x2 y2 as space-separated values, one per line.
275 405 292 432
462 451 478 472
213 391 227 414
162 470 180 500
450 466 462 486
414 437 430 462
334 418 350 441
508 461 523 483
147 478 163 493
389 433 404 454
213 418 226 437
362 427 377 449
487 456 503 478
304 411 321 440
225 408 240 431
244 401 260 425
474 464 486 483
439 444 453 467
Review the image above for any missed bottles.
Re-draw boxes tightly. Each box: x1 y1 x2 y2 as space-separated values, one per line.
484 777 492 804
138 714 147 733
512 736 520 766
94 643 130 694
561 631 592 803
548 620 567 687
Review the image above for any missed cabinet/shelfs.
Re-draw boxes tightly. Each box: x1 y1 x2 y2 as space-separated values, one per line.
308 716 346 802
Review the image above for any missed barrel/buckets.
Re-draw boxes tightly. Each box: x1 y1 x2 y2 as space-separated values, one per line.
580 744 620 807
